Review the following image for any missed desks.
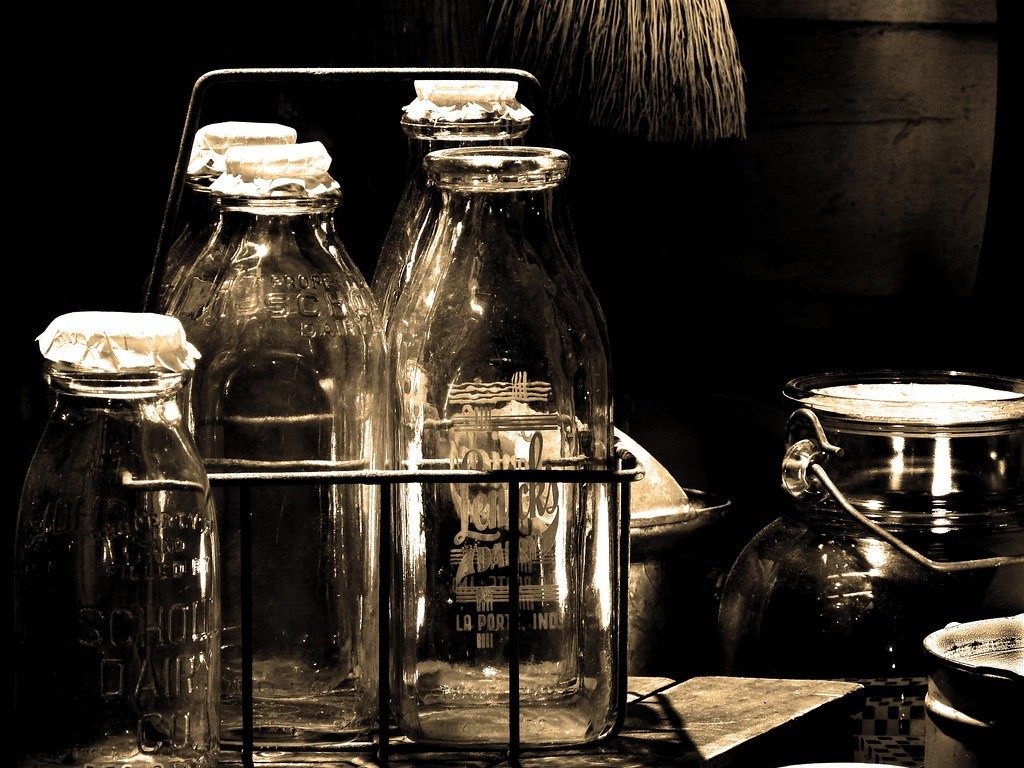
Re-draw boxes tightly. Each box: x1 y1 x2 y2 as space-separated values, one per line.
0 677 864 768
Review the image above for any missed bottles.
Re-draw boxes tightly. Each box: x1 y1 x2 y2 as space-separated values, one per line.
388 146 612 744
162 141 388 740
716 367 1023 768
370 80 589 708
158 121 297 312
13 312 219 767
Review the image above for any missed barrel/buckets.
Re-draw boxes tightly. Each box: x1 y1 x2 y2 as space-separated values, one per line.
923 613 1023 768
